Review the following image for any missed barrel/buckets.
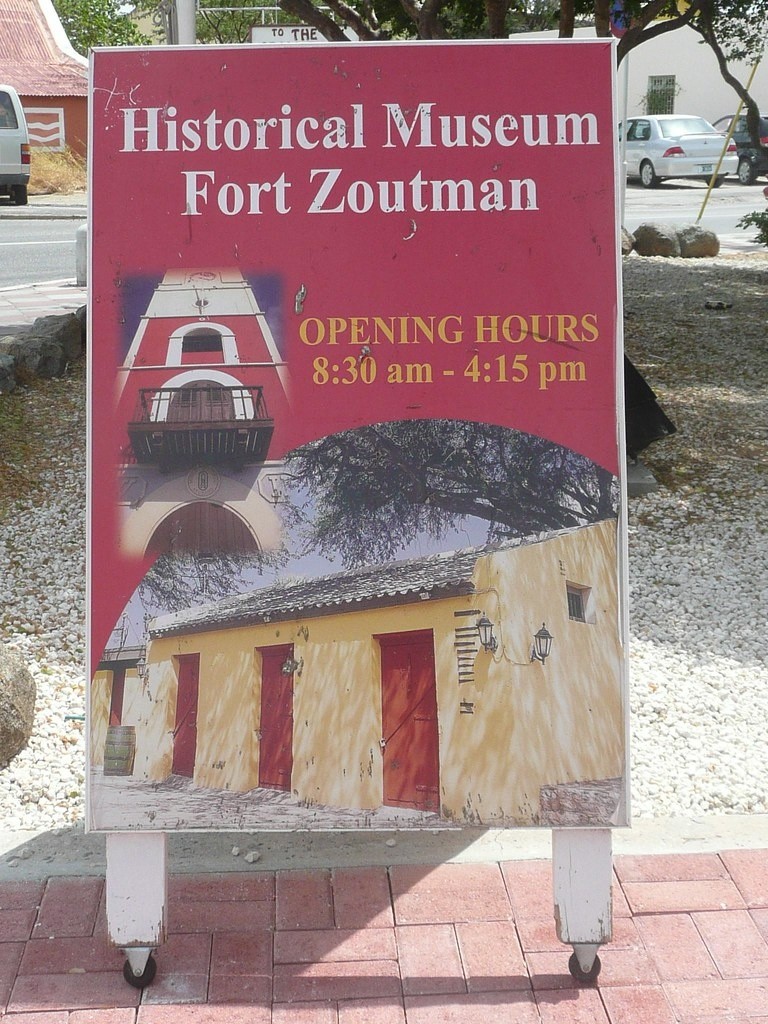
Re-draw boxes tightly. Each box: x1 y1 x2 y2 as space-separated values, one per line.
103 725 137 777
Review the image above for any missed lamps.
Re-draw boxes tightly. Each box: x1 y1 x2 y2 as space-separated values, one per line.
476 611 498 655
282 646 304 678
530 622 554 666
137 658 148 680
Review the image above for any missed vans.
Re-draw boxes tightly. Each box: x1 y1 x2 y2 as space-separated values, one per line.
0 83 32 206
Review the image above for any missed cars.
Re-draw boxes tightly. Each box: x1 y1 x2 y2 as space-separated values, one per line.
712 113 768 186
618 114 740 190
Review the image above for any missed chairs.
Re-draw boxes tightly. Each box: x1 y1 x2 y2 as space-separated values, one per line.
642 127 650 138
